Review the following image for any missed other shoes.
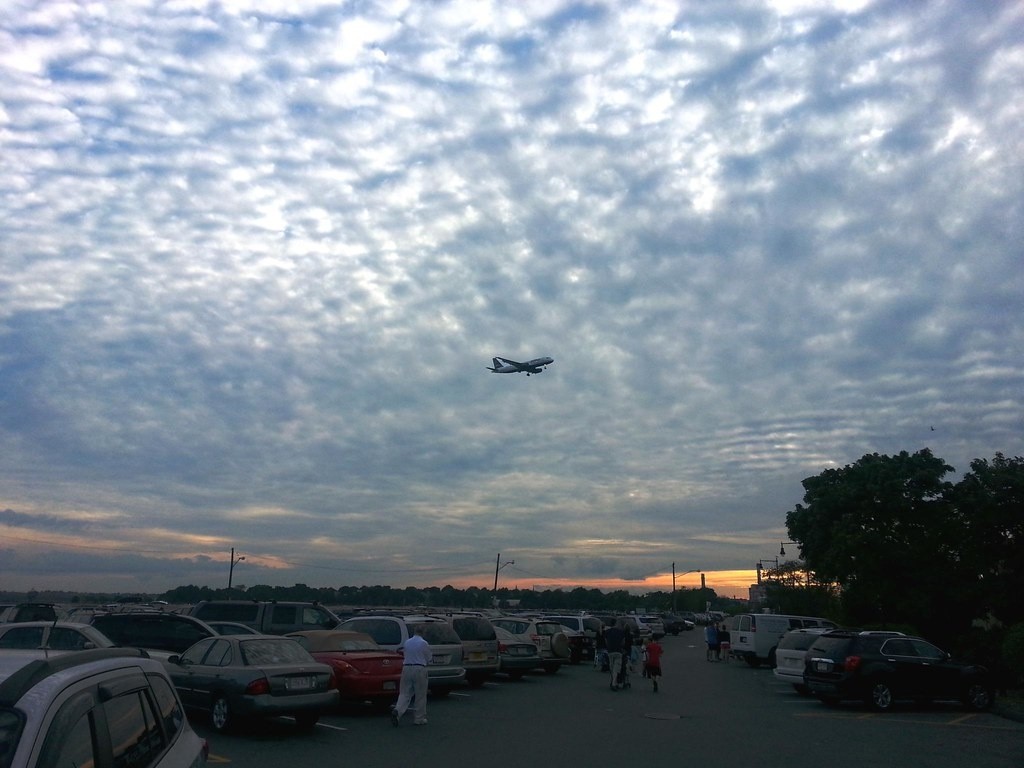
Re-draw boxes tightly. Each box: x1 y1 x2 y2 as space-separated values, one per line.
415 718 428 725
611 685 619 691
392 709 400 726
654 680 657 686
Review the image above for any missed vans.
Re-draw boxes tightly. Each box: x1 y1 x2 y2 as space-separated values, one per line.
803 630 992 713
730 613 840 669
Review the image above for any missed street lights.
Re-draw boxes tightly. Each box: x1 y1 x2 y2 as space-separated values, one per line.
780 541 810 610
495 560 514 596
672 562 700 612
758 559 780 592
229 557 245 589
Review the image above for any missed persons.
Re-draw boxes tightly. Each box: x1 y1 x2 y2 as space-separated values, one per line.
596 618 662 691
704 621 730 664
392 627 433 725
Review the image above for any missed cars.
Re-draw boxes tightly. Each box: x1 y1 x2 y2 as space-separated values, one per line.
773 627 833 693
1 596 729 768
289 630 404 707
164 633 339 734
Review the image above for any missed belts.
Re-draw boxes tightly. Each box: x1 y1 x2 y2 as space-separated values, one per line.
403 663 425 667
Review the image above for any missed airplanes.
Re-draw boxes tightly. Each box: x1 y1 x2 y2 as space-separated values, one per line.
486 356 555 376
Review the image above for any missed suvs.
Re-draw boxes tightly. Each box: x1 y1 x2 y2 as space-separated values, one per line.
332 609 465 695
